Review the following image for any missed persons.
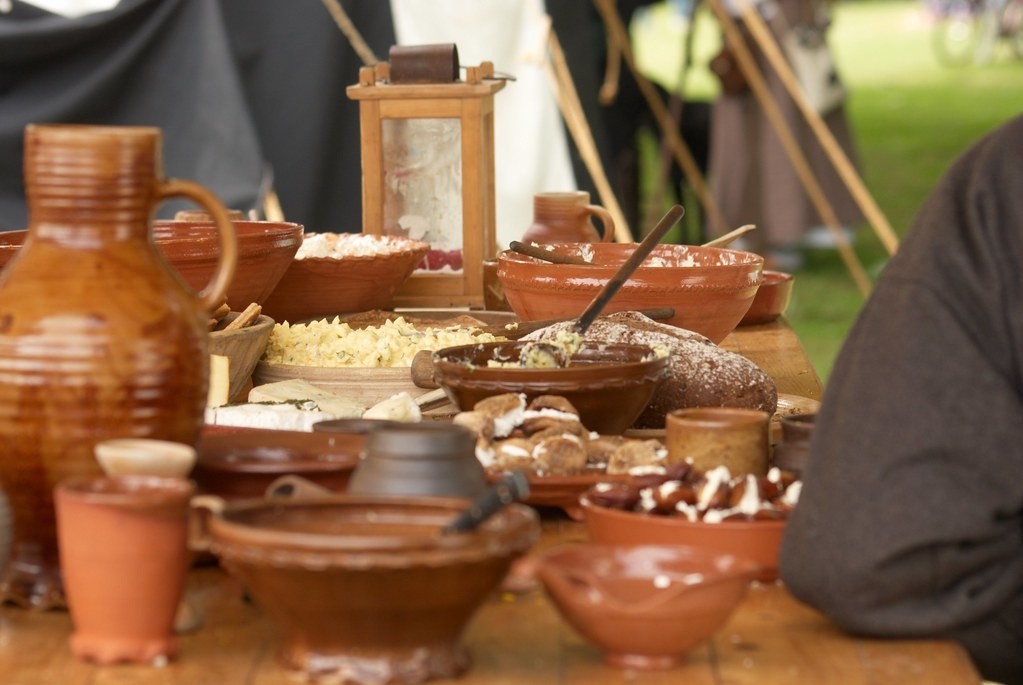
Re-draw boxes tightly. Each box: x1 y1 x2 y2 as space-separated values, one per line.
774 108 1023 684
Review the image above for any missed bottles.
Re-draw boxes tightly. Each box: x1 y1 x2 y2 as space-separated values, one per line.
774 413 815 483
0 122 237 615
342 421 498 507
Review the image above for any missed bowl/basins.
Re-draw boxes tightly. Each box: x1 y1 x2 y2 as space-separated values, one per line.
261 231 431 322
431 340 670 436
738 268 794 327
578 483 789 585
207 312 276 407
486 472 670 521
496 241 765 346
205 490 542 685
0 218 305 314
537 541 750 671
195 424 361 500
251 360 438 409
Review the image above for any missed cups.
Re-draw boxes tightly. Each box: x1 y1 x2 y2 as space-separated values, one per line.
520 189 616 244
53 474 195 663
665 407 770 480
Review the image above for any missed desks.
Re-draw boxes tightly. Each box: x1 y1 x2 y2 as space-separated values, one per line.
0 315 987 685
